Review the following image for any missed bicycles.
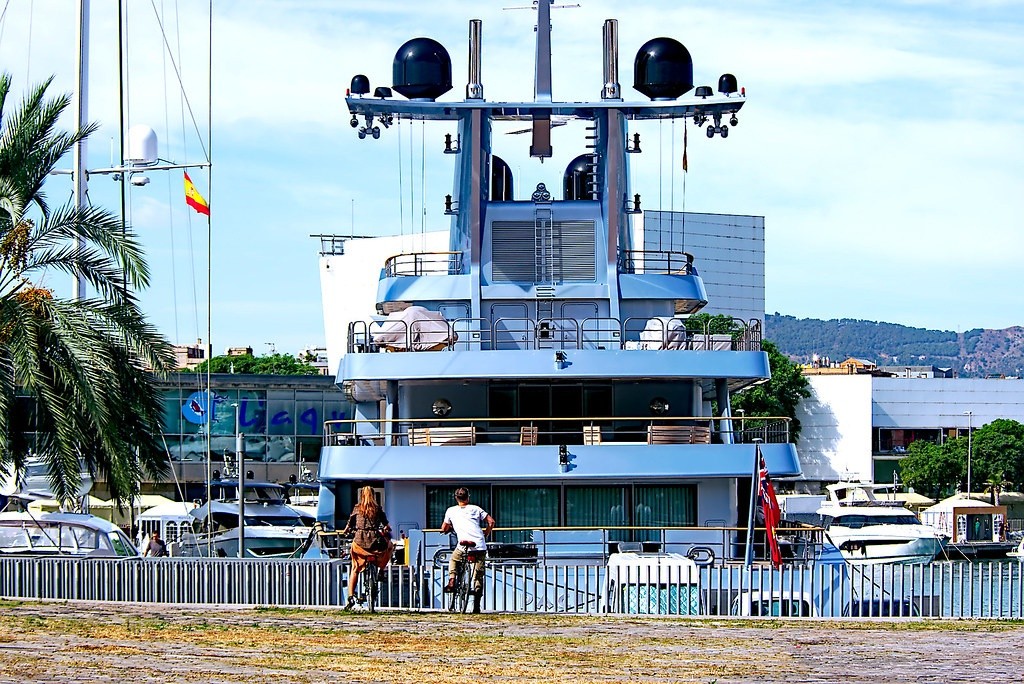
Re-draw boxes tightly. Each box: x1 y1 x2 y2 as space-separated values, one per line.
437 525 487 613
342 525 391 614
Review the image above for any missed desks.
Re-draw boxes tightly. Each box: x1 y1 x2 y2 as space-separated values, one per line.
693 334 732 350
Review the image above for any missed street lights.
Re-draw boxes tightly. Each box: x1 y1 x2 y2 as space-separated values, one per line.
963 411 972 501
230 402 240 476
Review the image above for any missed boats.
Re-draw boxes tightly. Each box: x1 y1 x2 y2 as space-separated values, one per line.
1 0 1023 617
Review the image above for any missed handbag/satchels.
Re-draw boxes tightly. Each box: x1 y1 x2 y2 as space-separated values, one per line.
367 533 397 570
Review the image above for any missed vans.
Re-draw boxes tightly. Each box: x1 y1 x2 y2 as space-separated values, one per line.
599 553 706 615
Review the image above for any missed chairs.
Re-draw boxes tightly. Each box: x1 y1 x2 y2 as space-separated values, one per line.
356 338 380 353
618 542 643 554
583 426 600 446
520 427 538 446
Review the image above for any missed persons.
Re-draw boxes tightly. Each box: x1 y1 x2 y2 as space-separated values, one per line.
343 486 393 610
998 520 1010 542
441 487 495 614
144 531 168 557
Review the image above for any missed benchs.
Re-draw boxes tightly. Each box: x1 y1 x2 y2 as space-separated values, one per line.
531 529 609 567
407 427 475 447
648 426 711 445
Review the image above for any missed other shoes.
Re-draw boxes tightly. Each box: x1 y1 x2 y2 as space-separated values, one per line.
343 597 356 610
377 569 386 580
473 606 482 613
443 578 457 593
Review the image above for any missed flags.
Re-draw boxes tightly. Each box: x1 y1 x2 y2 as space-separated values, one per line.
758 449 783 570
184 171 210 216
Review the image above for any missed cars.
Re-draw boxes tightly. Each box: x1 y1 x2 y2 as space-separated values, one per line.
729 591 819 617
844 600 922 617
